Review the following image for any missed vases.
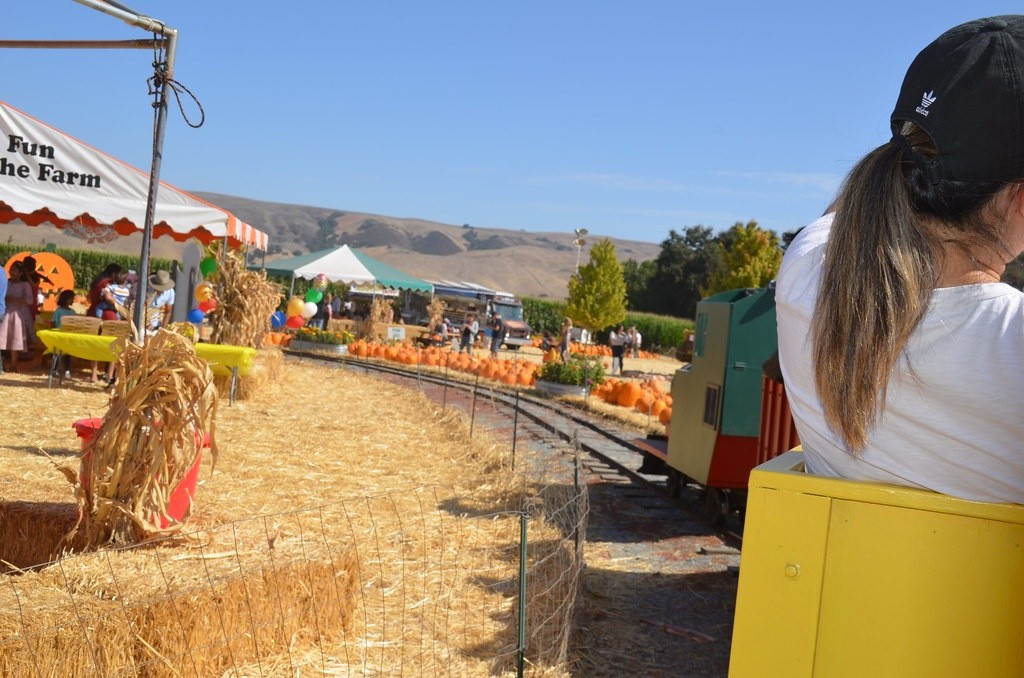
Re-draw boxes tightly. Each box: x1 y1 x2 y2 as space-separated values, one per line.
289 339 348 353
534 378 591 398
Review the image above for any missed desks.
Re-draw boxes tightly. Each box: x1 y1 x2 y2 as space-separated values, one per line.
34 311 86 349
36 328 255 407
419 330 461 343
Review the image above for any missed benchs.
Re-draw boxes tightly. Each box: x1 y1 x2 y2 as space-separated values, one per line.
728 444 1024 677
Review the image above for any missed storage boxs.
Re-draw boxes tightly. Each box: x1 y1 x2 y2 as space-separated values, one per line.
60 316 128 337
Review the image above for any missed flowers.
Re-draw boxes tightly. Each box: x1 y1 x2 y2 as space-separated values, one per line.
295 326 356 345
532 353 608 390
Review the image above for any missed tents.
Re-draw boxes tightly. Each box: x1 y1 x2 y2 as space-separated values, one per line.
246 243 434 323
0 101 269 275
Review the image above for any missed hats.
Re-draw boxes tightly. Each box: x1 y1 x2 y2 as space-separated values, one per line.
890 15 1024 182
148 270 174 291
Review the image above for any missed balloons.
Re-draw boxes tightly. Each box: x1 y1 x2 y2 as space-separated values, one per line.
188 282 218 324
270 274 327 332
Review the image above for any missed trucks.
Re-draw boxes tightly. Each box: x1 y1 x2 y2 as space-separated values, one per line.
434 285 533 351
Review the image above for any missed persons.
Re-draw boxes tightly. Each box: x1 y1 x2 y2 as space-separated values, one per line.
774 16 1024 509
309 291 504 359
0 256 175 383
543 317 643 376
